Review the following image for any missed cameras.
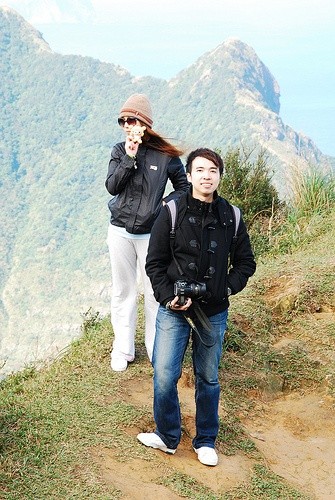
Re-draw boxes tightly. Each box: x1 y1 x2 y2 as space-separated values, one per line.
174 279 207 305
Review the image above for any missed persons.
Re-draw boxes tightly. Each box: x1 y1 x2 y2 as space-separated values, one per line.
105 94 186 371
137 149 256 466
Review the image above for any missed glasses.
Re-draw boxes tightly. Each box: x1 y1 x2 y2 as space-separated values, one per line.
118 117 145 127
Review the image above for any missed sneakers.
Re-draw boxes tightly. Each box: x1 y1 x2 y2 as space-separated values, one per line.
137 432 177 455
193 444 218 466
111 356 128 371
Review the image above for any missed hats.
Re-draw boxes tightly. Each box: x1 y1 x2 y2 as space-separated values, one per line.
119 93 154 129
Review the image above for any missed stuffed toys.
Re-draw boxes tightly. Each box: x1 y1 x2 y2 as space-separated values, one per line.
131 125 146 143
